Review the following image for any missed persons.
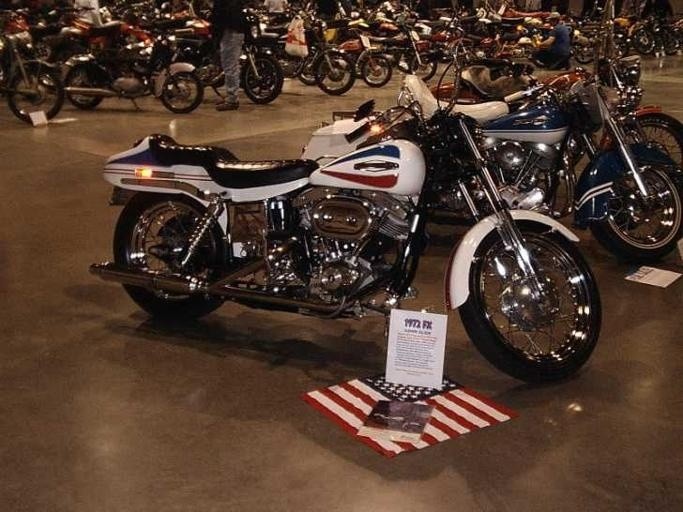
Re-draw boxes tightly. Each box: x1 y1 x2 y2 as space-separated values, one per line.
532 11 570 72
204 0 246 111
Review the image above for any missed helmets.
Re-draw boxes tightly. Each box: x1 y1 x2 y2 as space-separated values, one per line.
549 12 560 19
490 58 515 80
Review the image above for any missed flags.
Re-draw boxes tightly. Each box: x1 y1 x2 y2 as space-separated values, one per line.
298 371 520 460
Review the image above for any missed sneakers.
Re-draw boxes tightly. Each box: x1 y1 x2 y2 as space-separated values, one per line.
215 101 240 110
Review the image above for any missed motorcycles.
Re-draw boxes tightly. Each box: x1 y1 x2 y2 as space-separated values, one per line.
88 51 602 383
0 1 683 191
300 1 683 268
